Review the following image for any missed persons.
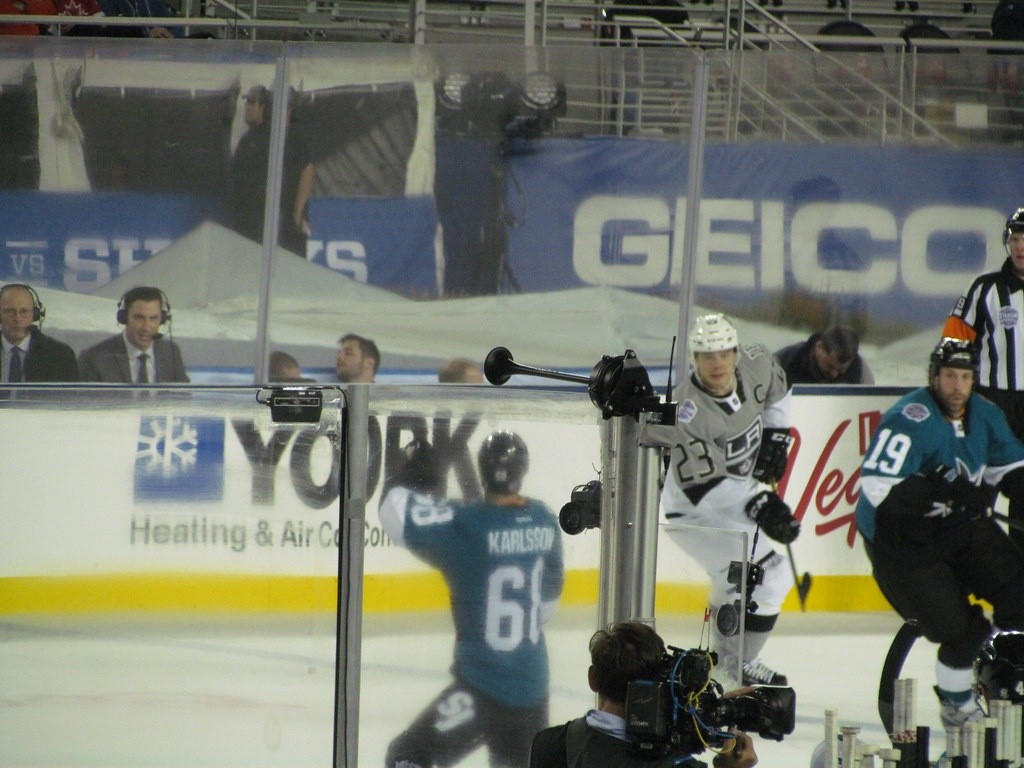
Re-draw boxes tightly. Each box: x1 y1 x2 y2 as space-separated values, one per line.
0 0 215 63
662 313 793 694
0 285 76 410
224 83 314 257
778 175 869 341
77 286 190 383
771 323 874 385
855 336 1024 734
942 208 1024 553
438 363 483 383
528 621 758 768
378 431 565 768
269 350 315 382
336 334 380 383
434 116 490 298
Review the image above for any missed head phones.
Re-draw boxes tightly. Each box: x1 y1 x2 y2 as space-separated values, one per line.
23 285 42 321
117 290 171 325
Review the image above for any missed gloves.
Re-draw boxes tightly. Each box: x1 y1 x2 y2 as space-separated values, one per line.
399 440 441 494
752 431 789 482
745 491 799 544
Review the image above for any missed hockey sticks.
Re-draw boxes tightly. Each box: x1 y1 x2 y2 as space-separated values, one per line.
769 473 812 603
825 678 1022 767
993 511 1024 532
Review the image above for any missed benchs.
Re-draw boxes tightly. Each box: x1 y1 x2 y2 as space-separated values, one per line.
0 0 1023 144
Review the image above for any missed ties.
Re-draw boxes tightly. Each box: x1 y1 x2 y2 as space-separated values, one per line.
137 354 150 384
9 346 22 383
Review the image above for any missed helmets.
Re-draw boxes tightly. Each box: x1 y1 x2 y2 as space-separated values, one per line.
931 337 980 370
1003 207 1024 235
477 431 529 493
689 314 739 354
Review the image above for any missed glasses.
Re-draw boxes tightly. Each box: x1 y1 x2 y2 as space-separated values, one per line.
4 310 34 317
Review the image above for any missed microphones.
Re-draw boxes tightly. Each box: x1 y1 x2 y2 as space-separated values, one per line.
28 316 45 333
152 322 170 340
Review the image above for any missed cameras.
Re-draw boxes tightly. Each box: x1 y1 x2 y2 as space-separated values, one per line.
559 481 602 535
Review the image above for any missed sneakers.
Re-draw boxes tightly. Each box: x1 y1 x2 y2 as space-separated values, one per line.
719 657 789 688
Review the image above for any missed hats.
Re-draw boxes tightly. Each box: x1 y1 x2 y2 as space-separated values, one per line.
242 86 270 105
